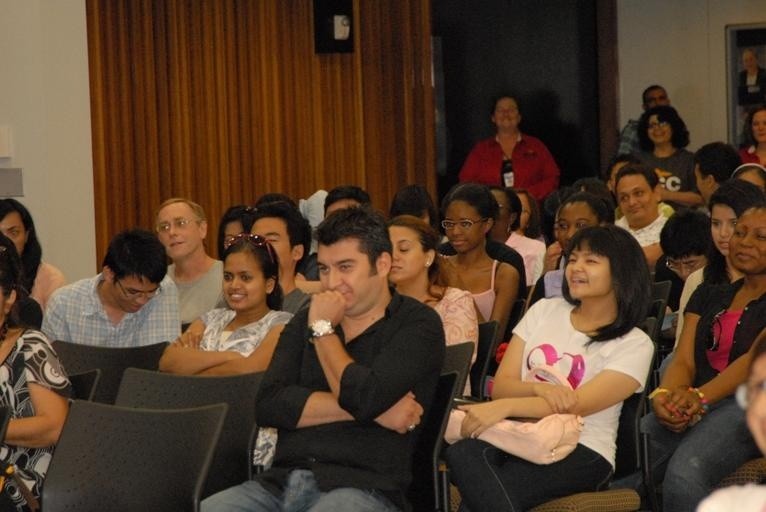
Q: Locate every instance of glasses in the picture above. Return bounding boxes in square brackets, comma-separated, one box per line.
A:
[441, 218, 485, 229]
[156, 218, 200, 233]
[666, 257, 707, 270]
[116, 277, 163, 301]
[228, 233, 273, 265]
[648, 120, 668, 129]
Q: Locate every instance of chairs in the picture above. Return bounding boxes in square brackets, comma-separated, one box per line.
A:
[0, 243, 766, 511]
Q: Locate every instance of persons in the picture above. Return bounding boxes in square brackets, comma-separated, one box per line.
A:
[0, 47, 766, 512]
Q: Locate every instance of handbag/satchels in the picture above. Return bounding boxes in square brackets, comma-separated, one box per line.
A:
[271, 420, 415, 491]
[443, 368, 584, 464]
